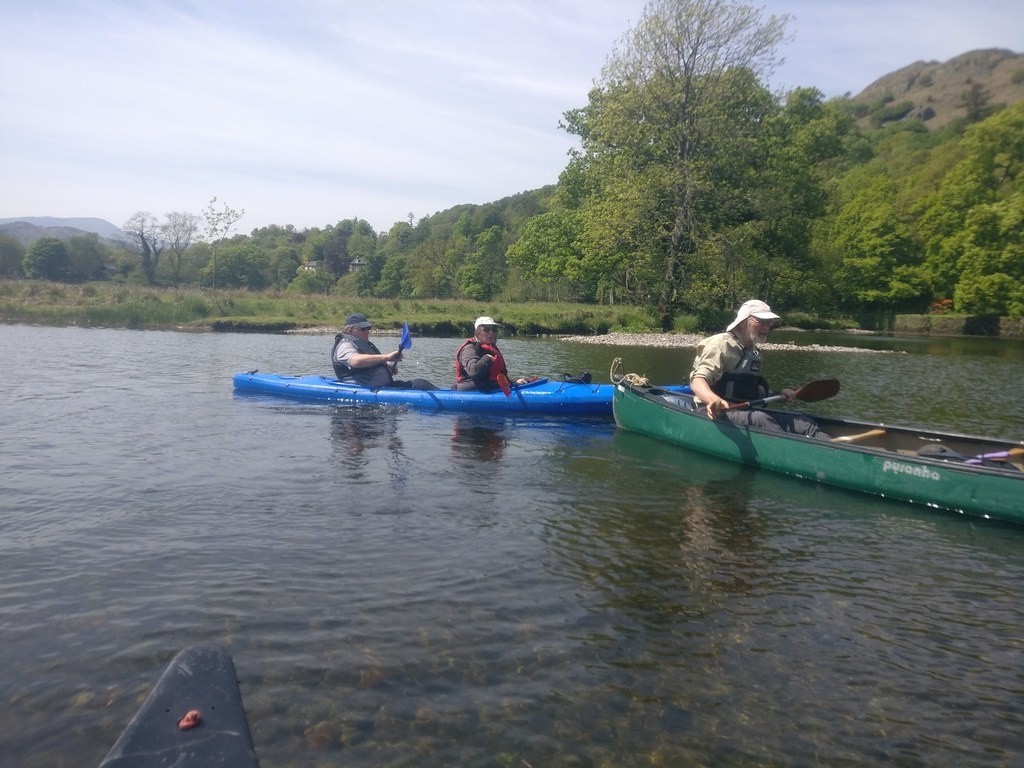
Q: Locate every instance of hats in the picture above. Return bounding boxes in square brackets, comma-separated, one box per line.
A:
[474, 316, 499, 329]
[727, 300, 780, 332]
[344, 313, 373, 329]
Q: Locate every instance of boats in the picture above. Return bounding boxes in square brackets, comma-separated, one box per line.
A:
[98, 642, 260, 767]
[233, 369, 692, 413]
[612, 379, 1024, 526]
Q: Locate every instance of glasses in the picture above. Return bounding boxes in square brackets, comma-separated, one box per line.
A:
[361, 326, 371, 331]
[748, 318, 775, 326]
[483, 326, 498, 333]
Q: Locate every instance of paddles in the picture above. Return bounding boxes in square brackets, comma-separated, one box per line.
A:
[721, 377, 841, 412]
[391, 319, 412, 371]
[496, 374, 539, 397]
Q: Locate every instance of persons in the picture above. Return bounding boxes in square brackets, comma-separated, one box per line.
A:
[331, 312, 442, 390]
[456, 316, 531, 389]
[690, 300, 835, 442]
